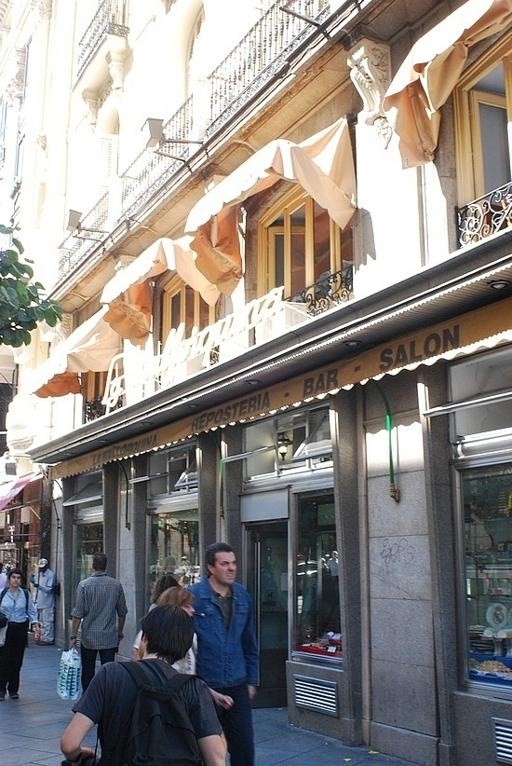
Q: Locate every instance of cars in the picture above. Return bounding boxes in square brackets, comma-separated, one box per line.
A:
[296, 560, 329, 596]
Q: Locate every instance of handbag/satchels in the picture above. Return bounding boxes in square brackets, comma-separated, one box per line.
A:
[57, 651, 83, 701]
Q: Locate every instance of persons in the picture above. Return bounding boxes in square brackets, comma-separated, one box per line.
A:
[0, 570, 43, 701]
[144, 574, 181, 610]
[184, 541, 260, 766]
[31, 558, 56, 646]
[5, 560, 12, 572]
[0, 563, 12, 591]
[68, 553, 128, 696]
[59, 606, 227, 766]
[319, 549, 339, 576]
[141, 587, 233, 766]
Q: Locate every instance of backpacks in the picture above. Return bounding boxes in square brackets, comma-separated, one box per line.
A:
[118, 661, 205, 766]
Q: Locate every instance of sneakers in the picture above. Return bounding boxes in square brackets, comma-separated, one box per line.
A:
[0, 692, 18, 701]
[40, 641, 54, 645]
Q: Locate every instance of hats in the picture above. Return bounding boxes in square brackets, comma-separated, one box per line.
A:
[38, 559, 48, 568]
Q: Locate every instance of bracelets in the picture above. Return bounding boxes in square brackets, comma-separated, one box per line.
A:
[71, 636, 77, 640]
